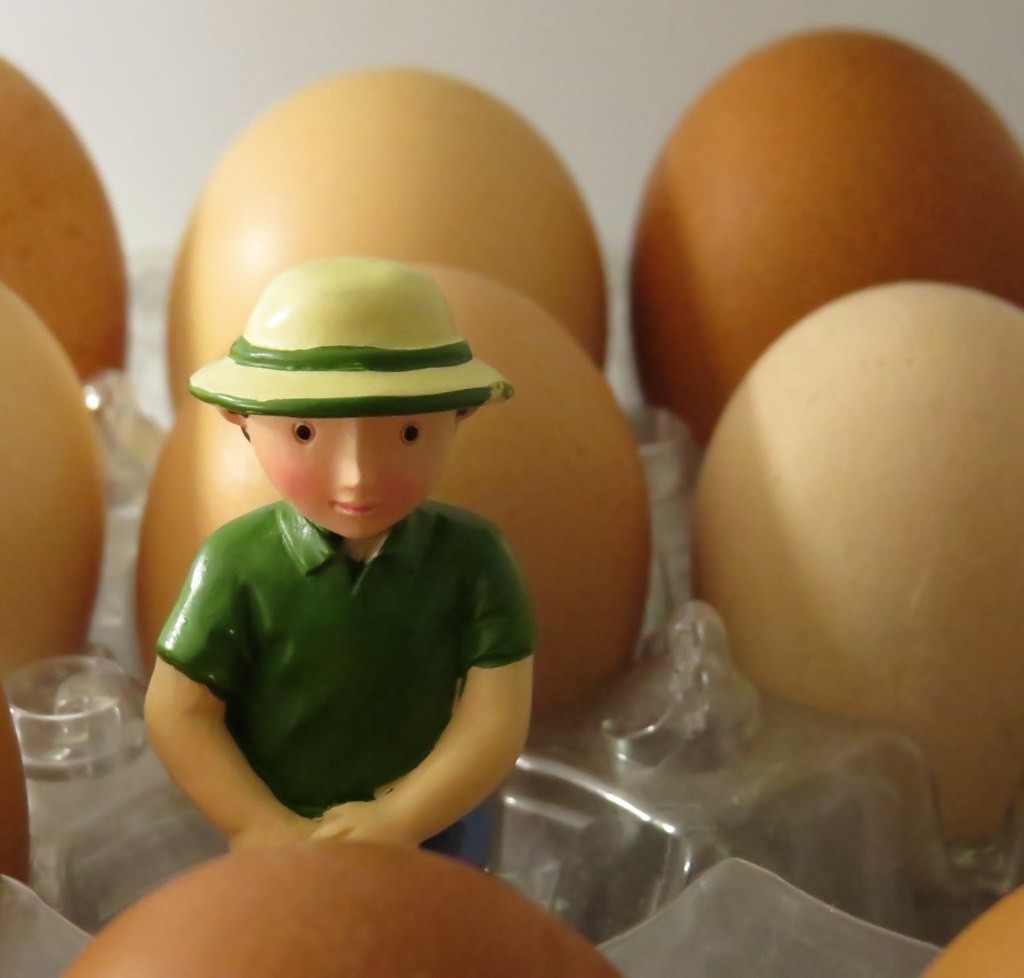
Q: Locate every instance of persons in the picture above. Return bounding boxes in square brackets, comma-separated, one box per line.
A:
[140, 253, 536, 870]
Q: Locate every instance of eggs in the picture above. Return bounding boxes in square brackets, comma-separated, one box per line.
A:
[0, 26, 1024, 978]
